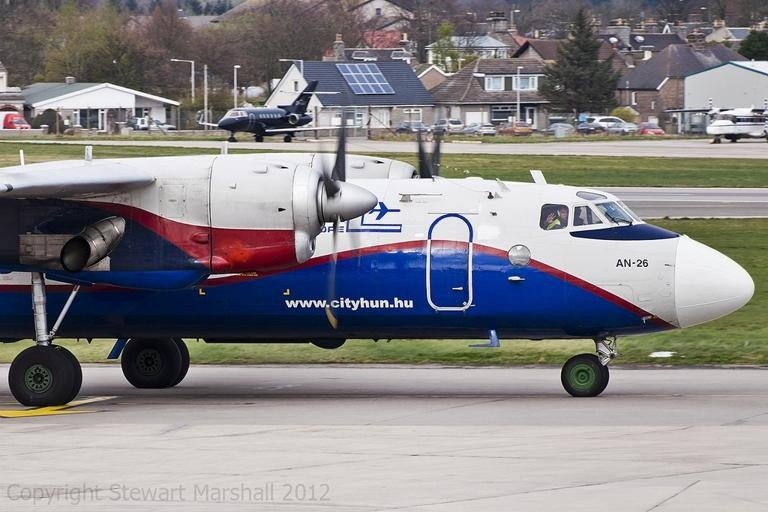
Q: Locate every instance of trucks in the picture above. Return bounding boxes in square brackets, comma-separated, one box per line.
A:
[0, 110, 31, 129]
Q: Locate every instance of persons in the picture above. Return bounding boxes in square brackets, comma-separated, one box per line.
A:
[541, 205, 569, 230]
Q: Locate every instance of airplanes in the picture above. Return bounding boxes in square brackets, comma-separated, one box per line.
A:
[705, 107, 768, 143]
[1, 115, 755, 406]
[198, 79, 362, 142]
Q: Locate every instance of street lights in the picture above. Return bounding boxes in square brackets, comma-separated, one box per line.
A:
[510, 9, 521, 25]
[170, 58, 195, 103]
[233, 65, 241, 108]
[517, 67, 525, 121]
[280, 58, 305, 77]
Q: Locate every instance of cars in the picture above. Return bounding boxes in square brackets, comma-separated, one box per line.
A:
[397, 119, 533, 137]
[542, 116, 664, 135]
[150, 119, 177, 130]
